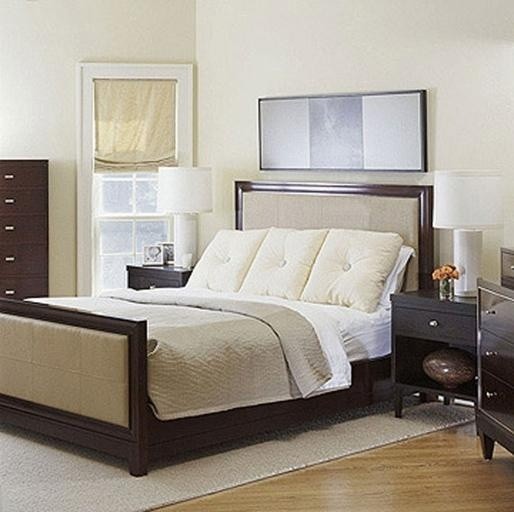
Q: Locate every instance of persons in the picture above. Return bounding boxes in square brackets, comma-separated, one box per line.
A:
[166, 244, 172, 261]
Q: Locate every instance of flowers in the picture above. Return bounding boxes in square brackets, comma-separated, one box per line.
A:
[432, 264, 462, 299]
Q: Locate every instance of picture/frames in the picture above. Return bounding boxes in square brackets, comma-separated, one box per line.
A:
[142, 242, 176, 266]
[257, 88, 427, 173]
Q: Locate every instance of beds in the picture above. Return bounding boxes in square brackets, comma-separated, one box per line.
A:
[1, 180, 434, 478]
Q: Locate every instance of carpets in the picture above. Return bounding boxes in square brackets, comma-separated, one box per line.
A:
[0, 386, 477, 511]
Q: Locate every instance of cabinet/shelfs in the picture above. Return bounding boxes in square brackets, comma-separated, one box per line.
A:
[0, 158, 48, 301]
[474, 247, 514, 459]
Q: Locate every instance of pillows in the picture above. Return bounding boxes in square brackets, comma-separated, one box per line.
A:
[237, 227, 329, 301]
[378, 245, 416, 311]
[185, 229, 267, 294]
[298, 228, 405, 313]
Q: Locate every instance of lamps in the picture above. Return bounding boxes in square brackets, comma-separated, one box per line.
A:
[157, 166, 213, 266]
[431, 171, 506, 298]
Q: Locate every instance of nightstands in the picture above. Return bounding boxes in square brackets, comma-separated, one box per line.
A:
[389, 286, 477, 419]
[125, 263, 193, 291]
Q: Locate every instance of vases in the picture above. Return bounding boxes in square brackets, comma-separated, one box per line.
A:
[439, 278, 454, 302]
